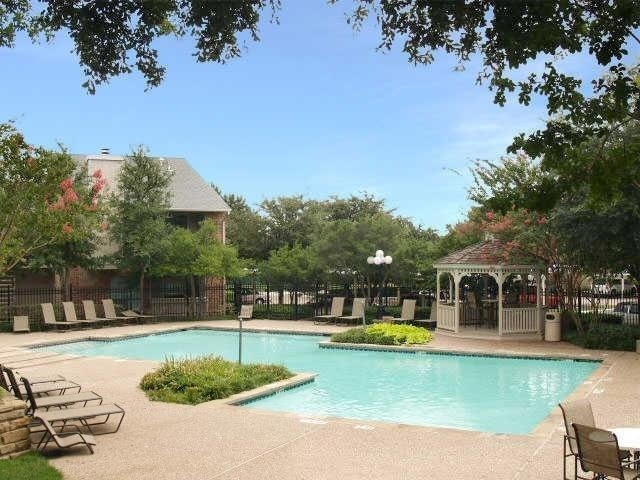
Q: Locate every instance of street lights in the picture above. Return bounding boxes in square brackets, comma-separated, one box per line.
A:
[367, 250, 393, 320]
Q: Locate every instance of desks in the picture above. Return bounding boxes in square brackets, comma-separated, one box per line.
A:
[593, 427, 640, 463]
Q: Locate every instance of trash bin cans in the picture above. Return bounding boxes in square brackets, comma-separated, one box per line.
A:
[544, 311, 561, 342]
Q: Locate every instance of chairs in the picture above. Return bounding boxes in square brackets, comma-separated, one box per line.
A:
[237, 303, 254, 321]
[568, 418, 640, 480]
[557, 398, 597, 480]
[0, 363, 129, 458]
[391, 297, 416, 324]
[311, 297, 348, 329]
[416, 300, 444, 331]
[452, 289, 498, 330]
[10, 296, 157, 336]
[339, 297, 370, 328]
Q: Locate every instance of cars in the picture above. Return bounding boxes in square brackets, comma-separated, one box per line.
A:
[418, 289, 435, 297]
[241, 287, 272, 305]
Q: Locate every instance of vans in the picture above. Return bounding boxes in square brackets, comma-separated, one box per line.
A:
[613, 301, 640, 326]
[591, 277, 637, 298]
